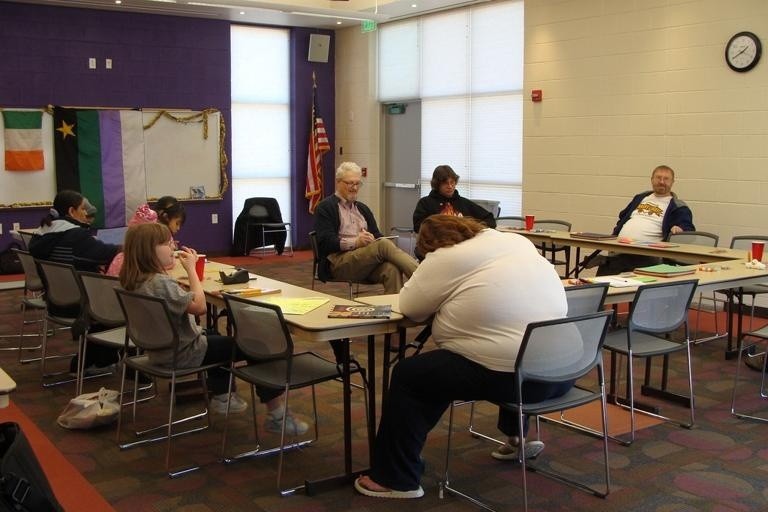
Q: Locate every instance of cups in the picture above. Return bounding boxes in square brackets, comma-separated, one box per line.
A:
[525, 215, 534, 232]
[751, 241, 765, 263]
[195, 254, 206, 282]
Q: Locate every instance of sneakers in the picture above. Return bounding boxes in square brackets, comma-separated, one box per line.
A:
[210, 393, 248, 413]
[264, 411, 308, 436]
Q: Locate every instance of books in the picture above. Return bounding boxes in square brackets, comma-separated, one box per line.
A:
[223, 288, 281, 298]
[634, 264, 697, 277]
[328, 304, 391, 319]
[571, 233, 618, 240]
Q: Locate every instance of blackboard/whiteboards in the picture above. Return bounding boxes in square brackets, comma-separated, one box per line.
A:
[0, 106, 222, 210]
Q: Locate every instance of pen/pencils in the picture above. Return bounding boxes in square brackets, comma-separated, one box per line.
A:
[361, 229, 374, 242]
[622, 276, 637, 279]
[225, 288, 265, 296]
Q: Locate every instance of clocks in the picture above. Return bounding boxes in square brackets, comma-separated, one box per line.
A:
[724, 31, 762, 73]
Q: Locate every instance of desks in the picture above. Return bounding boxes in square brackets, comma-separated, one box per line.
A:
[162, 252, 235, 395]
[173, 269, 403, 498]
[495, 227, 768, 361]
[356, 272, 660, 473]
[622, 260, 768, 409]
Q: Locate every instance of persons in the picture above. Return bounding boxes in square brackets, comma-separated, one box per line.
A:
[354, 215, 583, 498]
[119, 222, 309, 436]
[29, 190, 125, 373]
[596, 166, 695, 276]
[313, 162, 420, 296]
[107, 196, 185, 384]
[413, 165, 496, 262]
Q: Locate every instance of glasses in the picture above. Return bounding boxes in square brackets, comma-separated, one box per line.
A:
[340, 178, 363, 188]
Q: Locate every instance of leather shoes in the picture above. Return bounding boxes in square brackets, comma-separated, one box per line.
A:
[744, 356, 768, 372]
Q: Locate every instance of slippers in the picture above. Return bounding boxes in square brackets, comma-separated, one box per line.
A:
[491, 440, 544, 461]
[355, 476, 425, 498]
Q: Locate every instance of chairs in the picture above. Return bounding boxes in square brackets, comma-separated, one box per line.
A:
[692, 235, 768, 358]
[390, 226, 414, 257]
[442, 281, 615, 512]
[32, 258, 158, 406]
[731, 323, 768, 422]
[0, 230, 78, 363]
[494, 217, 572, 279]
[110, 286, 260, 477]
[307, 230, 397, 301]
[560, 278, 699, 445]
[662, 231, 719, 345]
[242, 197, 293, 260]
[219, 291, 371, 497]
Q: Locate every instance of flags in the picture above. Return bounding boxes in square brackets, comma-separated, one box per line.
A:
[52, 106, 147, 229]
[2, 111, 44, 171]
[305, 86, 331, 214]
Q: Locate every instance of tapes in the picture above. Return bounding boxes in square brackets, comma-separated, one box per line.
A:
[231, 271, 238, 277]
